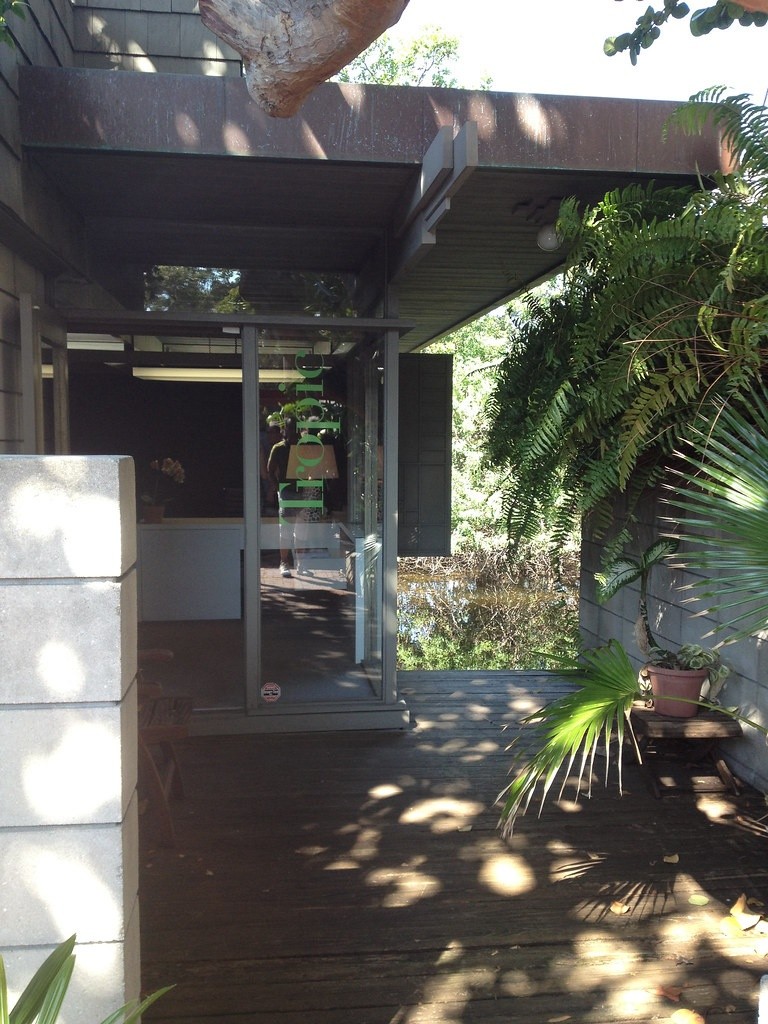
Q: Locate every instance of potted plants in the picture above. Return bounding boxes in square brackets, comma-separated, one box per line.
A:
[644, 642, 736, 717]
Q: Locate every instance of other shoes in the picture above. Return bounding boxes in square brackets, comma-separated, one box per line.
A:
[279, 563, 291, 577]
[297, 564, 304, 575]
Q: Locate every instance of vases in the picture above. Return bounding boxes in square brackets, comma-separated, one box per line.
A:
[142, 505, 165, 524]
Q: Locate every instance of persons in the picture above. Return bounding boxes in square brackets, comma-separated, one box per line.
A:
[267, 415, 341, 578]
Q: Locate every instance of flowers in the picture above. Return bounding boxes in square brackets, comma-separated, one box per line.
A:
[140, 458, 186, 506]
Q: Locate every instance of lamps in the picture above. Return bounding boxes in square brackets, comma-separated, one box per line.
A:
[285, 444, 339, 523]
